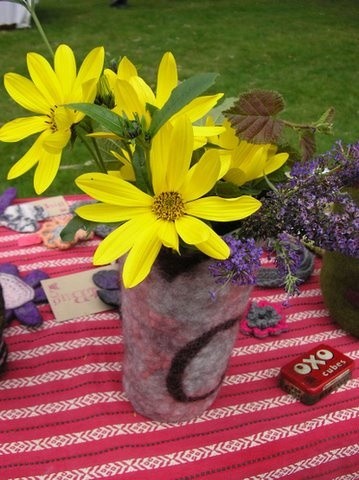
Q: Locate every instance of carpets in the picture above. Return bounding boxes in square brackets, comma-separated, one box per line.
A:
[0, 194, 359, 480]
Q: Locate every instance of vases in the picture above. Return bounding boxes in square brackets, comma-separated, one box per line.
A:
[320, 186, 359, 340]
[120, 259, 250, 423]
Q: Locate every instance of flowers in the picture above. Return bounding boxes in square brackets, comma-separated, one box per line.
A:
[208, 138, 359, 306]
[0, 0, 290, 289]
